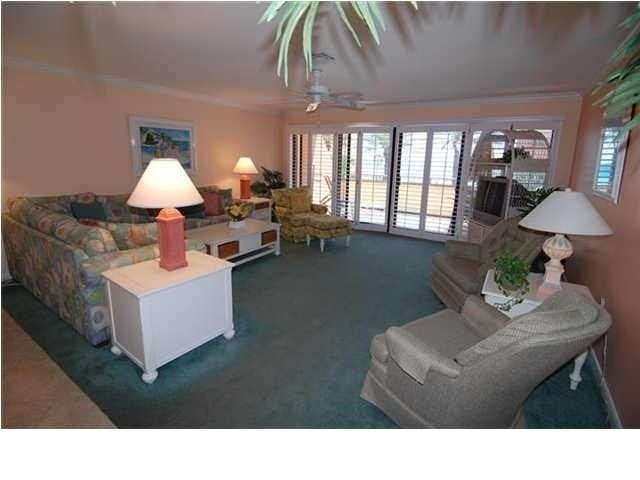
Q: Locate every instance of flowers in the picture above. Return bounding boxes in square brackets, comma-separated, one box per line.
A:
[223, 203, 250, 221]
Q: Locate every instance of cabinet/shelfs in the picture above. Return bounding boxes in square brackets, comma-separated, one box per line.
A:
[468, 218, 494, 244]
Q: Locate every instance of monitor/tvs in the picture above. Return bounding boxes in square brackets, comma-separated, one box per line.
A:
[472, 175, 529, 226]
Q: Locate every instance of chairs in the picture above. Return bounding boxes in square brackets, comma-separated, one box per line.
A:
[270, 185, 329, 244]
[430, 216, 546, 314]
[360, 288, 614, 428]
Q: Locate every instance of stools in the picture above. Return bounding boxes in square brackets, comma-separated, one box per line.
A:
[304, 215, 353, 253]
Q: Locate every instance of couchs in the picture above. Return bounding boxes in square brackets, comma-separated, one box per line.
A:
[1, 183, 233, 347]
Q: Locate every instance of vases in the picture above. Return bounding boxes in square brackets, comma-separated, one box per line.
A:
[228, 221, 245, 228]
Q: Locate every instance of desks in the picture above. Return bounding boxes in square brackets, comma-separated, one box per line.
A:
[99, 249, 237, 385]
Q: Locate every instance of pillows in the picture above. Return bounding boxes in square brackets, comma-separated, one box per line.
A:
[126, 191, 224, 219]
[70, 201, 107, 226]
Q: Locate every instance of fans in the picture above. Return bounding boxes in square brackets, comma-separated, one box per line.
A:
[281, 52, 367, 112]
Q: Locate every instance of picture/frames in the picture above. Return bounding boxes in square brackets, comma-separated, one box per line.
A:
[127, 114, 198, 177]
[592, 87, 635, 206]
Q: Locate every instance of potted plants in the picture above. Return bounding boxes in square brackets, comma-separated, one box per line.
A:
[250, 165, 288, 199]
[491, 246, 532, 313]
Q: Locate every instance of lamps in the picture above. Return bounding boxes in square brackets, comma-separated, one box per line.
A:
[519, 187, 615, 288]
[127, 158, 205, 271]
[232, 157, 259, 200]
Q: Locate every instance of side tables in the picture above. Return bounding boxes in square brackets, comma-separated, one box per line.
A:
[481, 268, 597, 392]
